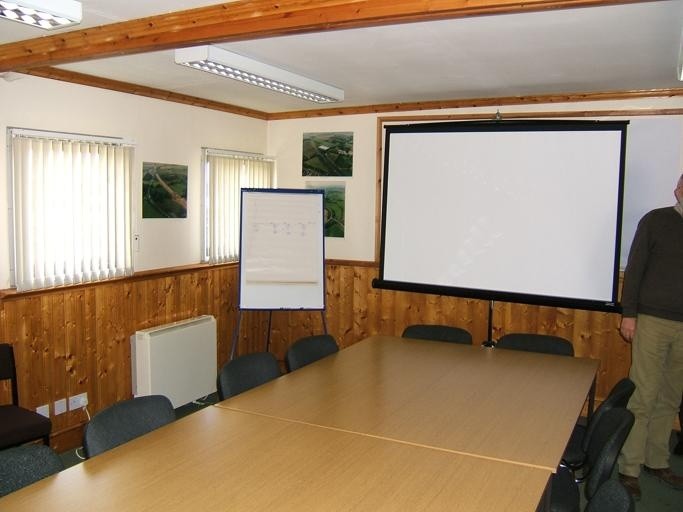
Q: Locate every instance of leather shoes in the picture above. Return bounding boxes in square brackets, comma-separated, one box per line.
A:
[643, 464, 683, 490]
[619, 469, 644, 502]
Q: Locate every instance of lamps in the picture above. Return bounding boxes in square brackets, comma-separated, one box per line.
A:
[173, 42, 345, 105]
[0, 0, 88, 34]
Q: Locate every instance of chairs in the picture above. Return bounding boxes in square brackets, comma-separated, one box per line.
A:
[540, 379, 635, 511]
[285, 334, 340, 373]
[2, 343, 52, 452]
[217, 351, 281, 401]
[81, 395, 176, 460]
[492, 332, 575, 357]
[2, 446, 66, 497]
[400, 324, 473, 346]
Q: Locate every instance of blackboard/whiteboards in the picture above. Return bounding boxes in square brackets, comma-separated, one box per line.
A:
[238, 188, 327, 312]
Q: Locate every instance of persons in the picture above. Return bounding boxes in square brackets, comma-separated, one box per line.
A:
[615, 172, 682, 499]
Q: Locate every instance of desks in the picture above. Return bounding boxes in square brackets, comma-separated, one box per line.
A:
[0, 335, 599, 512]
